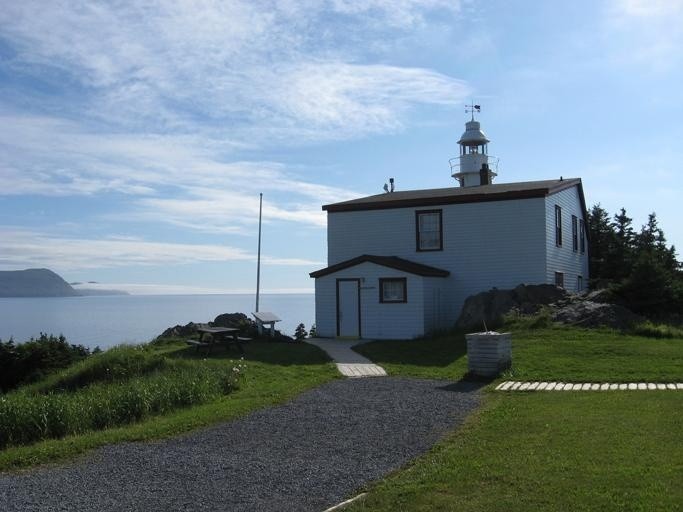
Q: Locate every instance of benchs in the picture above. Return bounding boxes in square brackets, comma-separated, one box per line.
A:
[186, 339, 210, 354]
[225, 335, 252, 349]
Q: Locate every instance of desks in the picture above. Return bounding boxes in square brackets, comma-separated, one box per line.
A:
[197, 327, 241, 352]
